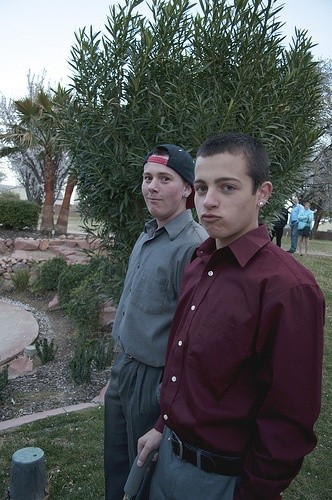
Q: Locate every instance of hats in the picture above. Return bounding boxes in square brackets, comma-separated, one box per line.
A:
[143, 144, 195, 208]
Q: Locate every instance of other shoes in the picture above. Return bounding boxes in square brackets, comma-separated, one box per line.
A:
[286, 250, 295, 253]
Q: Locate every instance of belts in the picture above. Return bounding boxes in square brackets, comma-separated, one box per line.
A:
[168, 430, 257, 476]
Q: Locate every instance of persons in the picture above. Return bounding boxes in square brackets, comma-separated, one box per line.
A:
[286, 197, 304, 253]
[103, 143, 207, 500]
[297, 202, 314, 255]
[269, 207, 288, 248]
[136, 132, 325, 500]
